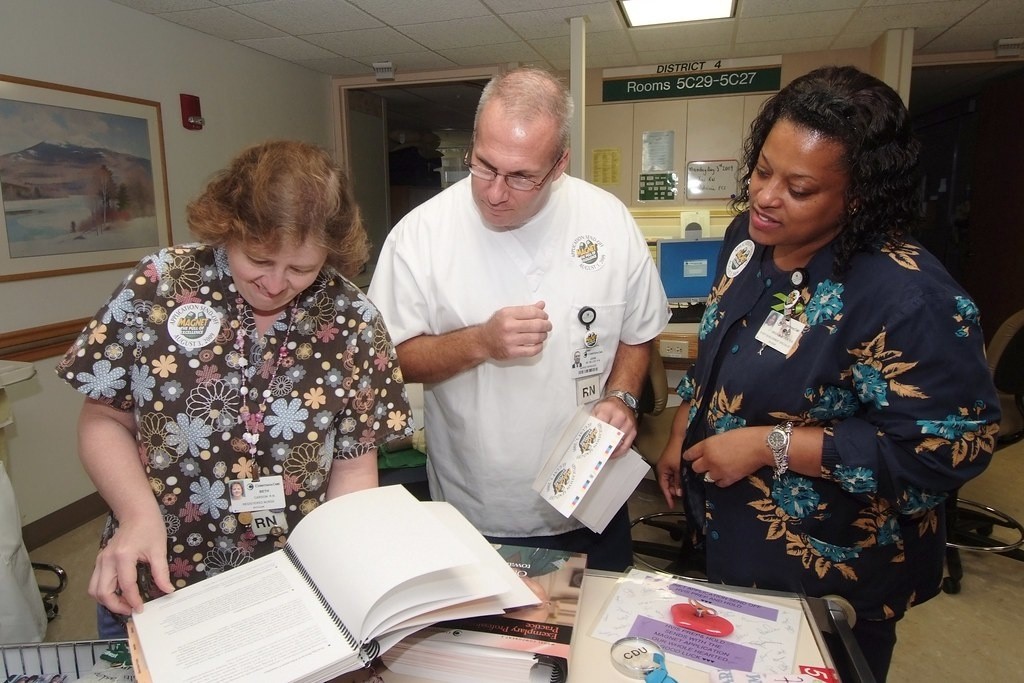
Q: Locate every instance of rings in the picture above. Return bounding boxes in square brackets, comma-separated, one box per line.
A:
[703, 472, 715, 482]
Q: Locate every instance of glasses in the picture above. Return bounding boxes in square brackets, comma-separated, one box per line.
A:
[464, 142, 564, 190]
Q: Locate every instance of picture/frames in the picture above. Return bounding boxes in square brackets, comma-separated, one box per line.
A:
[0, 74, 174, 283]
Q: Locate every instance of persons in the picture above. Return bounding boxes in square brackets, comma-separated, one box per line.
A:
[55, 139, 416, 640]
[765, 313, 778, 327]
[654, 64, 1004, 683]
[496, 545, 572, 624]
[230, 482, 244, 500]
[572, 351, 582, 368]
[367, 62, 674, 573]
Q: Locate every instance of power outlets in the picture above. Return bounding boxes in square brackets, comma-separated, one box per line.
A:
[659, 339, 689, 358]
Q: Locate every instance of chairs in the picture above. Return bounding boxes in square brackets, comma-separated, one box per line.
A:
[943, 311, 1024, 596]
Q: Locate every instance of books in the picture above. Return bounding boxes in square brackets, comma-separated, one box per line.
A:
[127, 483, 541, 682]
[379, 541, 589, 682]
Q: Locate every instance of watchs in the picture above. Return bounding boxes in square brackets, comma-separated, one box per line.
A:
[605, 389, 640, 413]
[766, 420, 793, 476]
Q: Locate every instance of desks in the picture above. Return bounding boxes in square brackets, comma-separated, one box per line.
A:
[586, 567, 872, 683]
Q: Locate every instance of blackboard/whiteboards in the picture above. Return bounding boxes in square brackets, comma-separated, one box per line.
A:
[686, 159, 740, 200]
